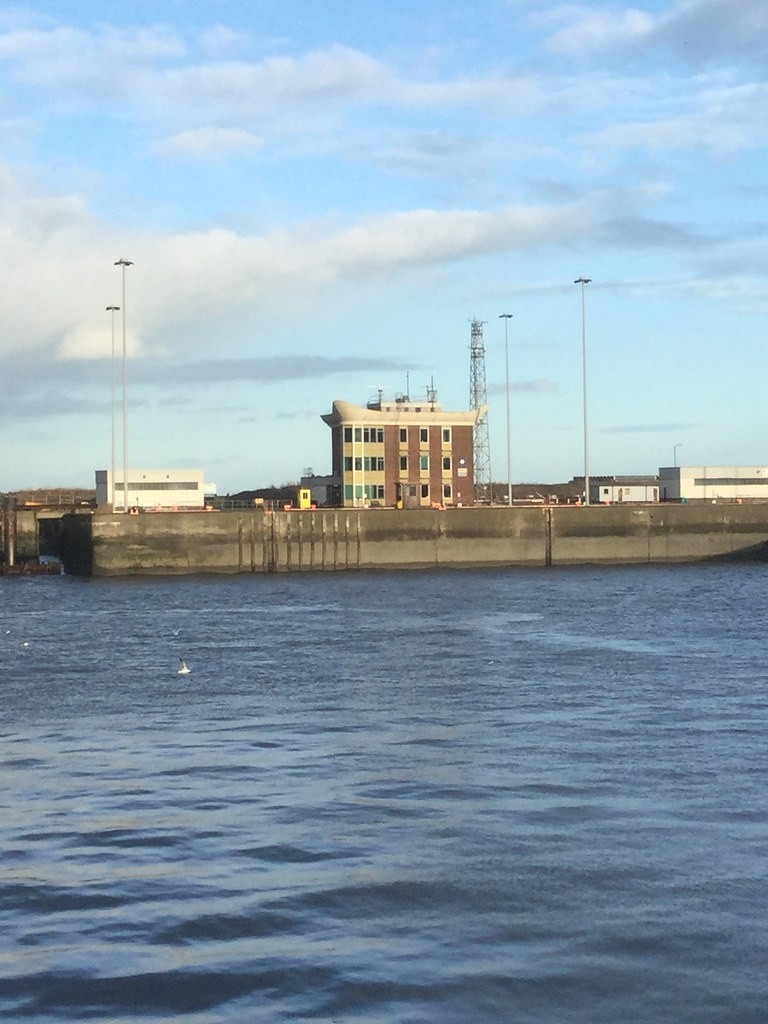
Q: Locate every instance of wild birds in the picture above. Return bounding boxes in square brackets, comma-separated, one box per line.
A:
[178, 658, 190, 675]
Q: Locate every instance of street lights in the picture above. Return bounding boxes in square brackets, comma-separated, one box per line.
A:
[106, 304, 120, 514]
[114, 256, 136, 515]
[673, 443, 683, 467]
[575, 276, 592, 506]
[498, 313, 515, 505]
